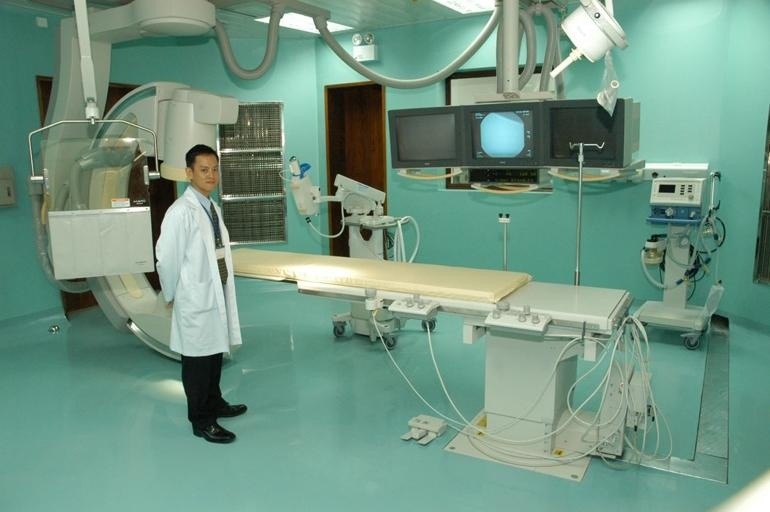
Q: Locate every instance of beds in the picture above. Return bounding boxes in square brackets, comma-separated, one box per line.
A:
[231, 247, 532, 316]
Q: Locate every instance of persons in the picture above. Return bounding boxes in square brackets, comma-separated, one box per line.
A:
[155, 144, 247, 443]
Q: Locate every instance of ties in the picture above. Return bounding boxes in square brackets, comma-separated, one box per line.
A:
[210, 201, 228, 285]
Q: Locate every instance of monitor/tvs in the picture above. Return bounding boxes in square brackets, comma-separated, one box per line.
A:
[388, 106, 466, 169]
[465, 102, 545, 169]
[544, 97, 640, 170]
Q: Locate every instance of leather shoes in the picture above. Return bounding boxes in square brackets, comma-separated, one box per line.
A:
[216, 405, 247, 417]
[193, 422, 235, 443]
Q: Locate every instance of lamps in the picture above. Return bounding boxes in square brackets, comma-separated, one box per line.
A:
[348, 32, 377, 62]
